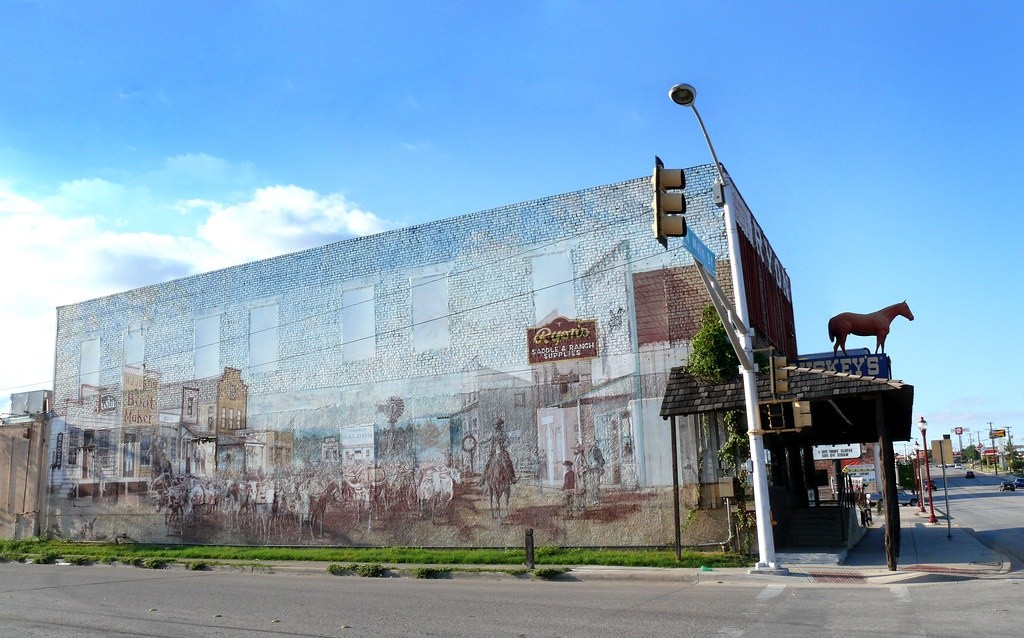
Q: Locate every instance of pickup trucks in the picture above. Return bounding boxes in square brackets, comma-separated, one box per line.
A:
[867, 487, 919, 508]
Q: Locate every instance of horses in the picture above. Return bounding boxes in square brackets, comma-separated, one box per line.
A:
[827, 299, 915, 357]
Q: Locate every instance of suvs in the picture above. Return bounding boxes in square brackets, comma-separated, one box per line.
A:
[924, 480, 937, 492]
[966, 471, 974, 479]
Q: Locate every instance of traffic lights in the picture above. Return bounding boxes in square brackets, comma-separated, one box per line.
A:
[650, 155, 691, 252]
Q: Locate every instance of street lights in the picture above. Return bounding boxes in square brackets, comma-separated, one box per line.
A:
[918, 416, 941, 526]
[667, 82, 790, 576]
[910, 451, 919, 495]
[914, 441, 927, 513]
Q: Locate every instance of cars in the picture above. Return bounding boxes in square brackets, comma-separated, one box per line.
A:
[955, 463, 962, 469]
[999, 481, 1015, 492]
[1015, 479, 1024, 489]
[937, 463, 955, 467]
